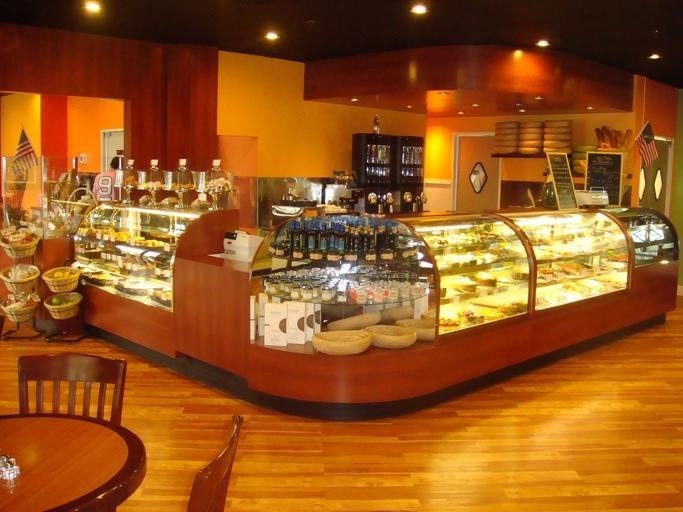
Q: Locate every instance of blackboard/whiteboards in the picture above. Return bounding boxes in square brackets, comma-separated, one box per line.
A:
[546, 152, 579, 211]
[584, 151, 623, 208]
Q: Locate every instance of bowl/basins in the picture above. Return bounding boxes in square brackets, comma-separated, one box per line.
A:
[0, 231, 82, 323]
[494, 120, 572, 156]
[311, 304, 437, 355]
[571, 145, 597, 174]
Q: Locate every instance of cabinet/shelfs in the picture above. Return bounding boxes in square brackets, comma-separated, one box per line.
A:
[73, 194, 241, 367]
[589, 202, 679, 335]
[484, 198, 634, 363]
[258, 215, 425, 413]
[352, 130, 423, 190]
[404, 210, 532, 392]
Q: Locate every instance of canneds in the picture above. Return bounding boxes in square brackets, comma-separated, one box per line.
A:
[262, 274, 340, 303]
[269, 240, 420, 265]
[79, 231, 138, 272]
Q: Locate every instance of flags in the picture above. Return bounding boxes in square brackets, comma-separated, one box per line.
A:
[636, 122, 659, 169]
[11, 128, 40, 176]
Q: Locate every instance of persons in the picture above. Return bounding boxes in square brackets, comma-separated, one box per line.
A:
[92, 157, 123, 202]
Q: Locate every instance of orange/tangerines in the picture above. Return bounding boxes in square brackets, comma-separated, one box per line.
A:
[52, 298, 60, 304]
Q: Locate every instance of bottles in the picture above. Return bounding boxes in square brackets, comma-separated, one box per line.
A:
[287, 216, 400, 251]
[366, 144, 422, 165]
[0, 451, 21, 486]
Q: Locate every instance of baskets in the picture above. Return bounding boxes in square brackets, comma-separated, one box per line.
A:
[326, 310, 381, 331]
[397, 316, 436, 341]
[381, 303, 415, 324]
[41, 265, 79, 293]
[1, 228, 41, 259]
[43, 292, 83, 320]
[1, 264, 39, 292]
[365, 325, 415, 349]
[422, 309, 437, 320]
[0, 292, 39, 323]
[313, 329, 372, 354]
[494, 119, 574, 156]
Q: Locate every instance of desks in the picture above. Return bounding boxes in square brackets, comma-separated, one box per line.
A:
[2, 413, 149, 512]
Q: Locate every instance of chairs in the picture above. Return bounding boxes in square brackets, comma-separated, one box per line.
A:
[187, 412, 244, 512]
[19, 351, 129, 426]
